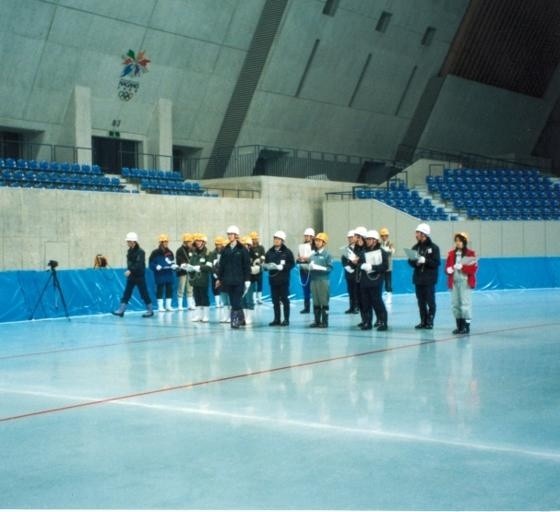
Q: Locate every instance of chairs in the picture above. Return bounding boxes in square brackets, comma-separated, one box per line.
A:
[123, 166, 220, 197]
[424, 166, 560, 220]
[1, 156, 138, 194]
[353, 179, 458, 222]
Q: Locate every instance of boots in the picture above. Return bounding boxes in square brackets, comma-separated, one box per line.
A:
[112, 304, 127, 317]
[157, 299, 166, 311]
[358, 311, 365, 327]
[142, 303, 153, 316]
[258, 292, 264, 304]
[217, 305, 227, 322]
[374, 308, 381, 328]
[252, 292, 258, 305]
[311, 304, 322, 327]
[353, 296, 359, 313]
[269, 304, 281, 325]
[426, 303, 435, 329]
[190, 306, 202, 322]
[282, 305, 289, 326]
[215, 295, 220, 308]
[322, 305, 329, 328]
[231, 310, 239, 329]
[345, 295, 353, 313]
[415, 308, 426, 328]
[178, 297, 185, 310]
[452, 318, 462, 334]
[201, 306, 210, 322]
[219, 295, 223, 307]
[187, 296, 196, 309]
[384, 293, 392, 306]
[361, 309, 372, 330]
[238, 310, 245, 326]
[225, 307, 231, 322]
[377, 311, 387, 330]
[460, 319, 470, 334]
[166, 298, 174, 311]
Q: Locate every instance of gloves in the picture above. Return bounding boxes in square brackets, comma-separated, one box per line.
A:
[311, 265, 326, 271]
[187, 264, 194, 272]
[361, 263, 372, 272]
[156, 264, 162, 271]
[275, 265, 284, 270]
[244, 280, 252, 288]
[416, 261, 421, 266]
[308, 261, 314, 269]
[124, 270, 131, 276]
[447, 267, 454, 274]
[345, 265, 355, 273]
[181, 262, 188, 270]
[454, 264, 463, 270]
[193, 265, 201, 273]
[171, 264, 178, 270]
[253, 258, 260, 266]
[418, 256, 425, 263]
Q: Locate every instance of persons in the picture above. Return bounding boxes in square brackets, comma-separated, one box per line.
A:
[307, 232, 334, 328]
[176, 225, 266, 328]
[111, 232, 154, 318]
[262, 230, 296, 327]
[379, 227, 395, 292]
[352, 226, 381, 327]
[340, 229, 359, 315]
[444, 230, 479, 335]
[295, 226, 316, 314]
[352, 229, 390, 332]
[148, 232, 179, 312]
[407, 222, 441, 329]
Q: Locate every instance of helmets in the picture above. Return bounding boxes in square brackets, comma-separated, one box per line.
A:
[354, 227, 367, 236]
[240, 236, 253, 245]
[304, 228, 315, 236]
[380, 228, 388, 236]
[159, 233, 169, 241]
[181, 233, 192, 241]
[195, 234, 208, 242]
[313, 233, 329, 242]
[125, 232, 138, 241]
[273, 231, 287, 241]
[227, 225, 240, 235]
[415, 223, 431, 235]
[215, 236, 225, 245]
[348, 230, 354, 237]
[249, 231, 258, 239]
[365, 230, 380, 239]
[192, 232, 201, 240]
[454, 230, 468, 240]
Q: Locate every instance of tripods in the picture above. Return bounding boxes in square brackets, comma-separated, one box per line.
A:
[28, 272, 70, 322]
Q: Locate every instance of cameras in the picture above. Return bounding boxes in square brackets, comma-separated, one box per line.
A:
[48, 260, 58, 271]
